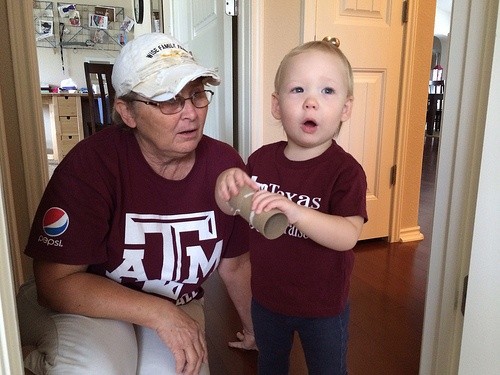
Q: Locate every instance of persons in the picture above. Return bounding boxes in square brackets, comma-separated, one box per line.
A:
[214, 40, 367, 375]
[16, 34, 257, 374]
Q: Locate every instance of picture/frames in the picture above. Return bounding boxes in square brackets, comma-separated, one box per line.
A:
[150, 0, 164, 33]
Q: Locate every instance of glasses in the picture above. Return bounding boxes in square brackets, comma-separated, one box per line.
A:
[131, 90, 214, 115]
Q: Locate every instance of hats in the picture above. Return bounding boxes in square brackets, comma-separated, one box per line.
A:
[112, 32, 221, 101]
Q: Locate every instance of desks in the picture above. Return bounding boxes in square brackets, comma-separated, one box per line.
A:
[42, 92, 103, 164]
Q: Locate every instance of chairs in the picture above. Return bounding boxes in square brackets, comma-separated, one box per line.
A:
[84, 62, 113, 134]
[427, 79, 443, 133]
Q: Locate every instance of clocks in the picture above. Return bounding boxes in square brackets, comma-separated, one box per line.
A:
[135, 0, 143, 23]
